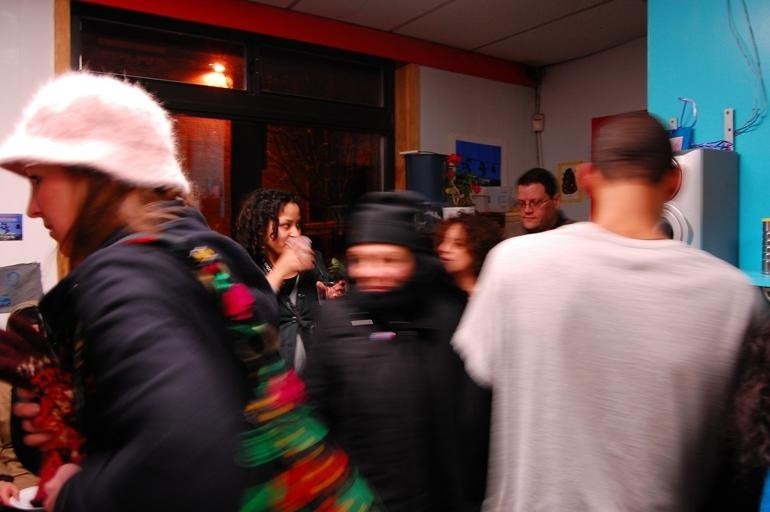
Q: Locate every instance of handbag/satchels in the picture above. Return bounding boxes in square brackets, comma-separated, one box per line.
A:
[236, 368, 387, 512]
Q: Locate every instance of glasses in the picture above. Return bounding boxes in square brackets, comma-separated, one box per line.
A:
[513, 197, 552, 210]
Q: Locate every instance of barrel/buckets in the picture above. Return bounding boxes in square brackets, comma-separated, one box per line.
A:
[400, 149, 447, 202]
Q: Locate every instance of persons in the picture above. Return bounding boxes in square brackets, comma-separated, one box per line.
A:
[237, 189, 346, 380]
[0, 462, 21, 508]
[507, 168, 576, 238]
[450, 113, 770, 512]
[301, 190, 489, 512]
[436, 211, 504, 300]
[0, 69, 389, 511]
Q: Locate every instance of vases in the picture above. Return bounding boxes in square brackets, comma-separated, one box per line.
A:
[442, 208, 475, 223]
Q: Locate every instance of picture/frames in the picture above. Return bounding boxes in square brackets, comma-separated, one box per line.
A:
[557, 161, 585, 204]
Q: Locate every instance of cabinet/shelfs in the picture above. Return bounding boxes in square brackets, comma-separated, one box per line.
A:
[481, 213, 527, 238]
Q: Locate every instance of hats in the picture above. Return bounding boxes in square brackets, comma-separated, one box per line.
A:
[1, 71, 189, 197]
[341, 192, 442, 256]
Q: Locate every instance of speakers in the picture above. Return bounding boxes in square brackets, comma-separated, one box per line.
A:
[659, 148, 740, 270]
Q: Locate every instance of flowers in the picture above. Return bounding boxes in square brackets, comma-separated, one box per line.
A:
[443, 153, 481, 207]
